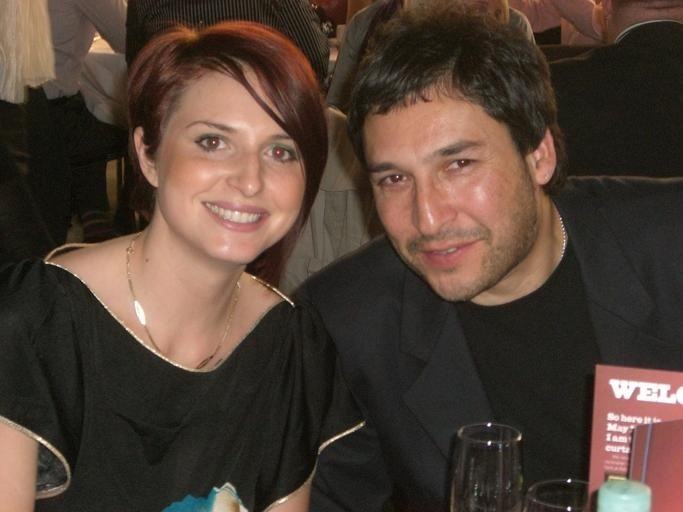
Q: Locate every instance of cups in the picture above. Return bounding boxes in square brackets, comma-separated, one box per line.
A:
[448, 423, 524, 512]
[523, 477, 597, 512]
